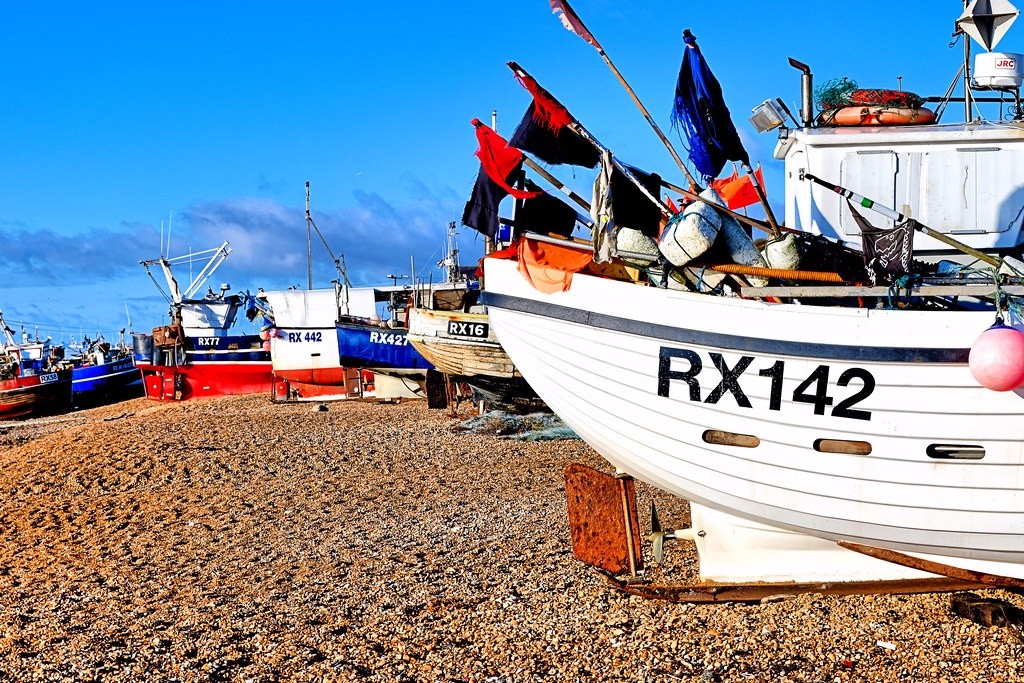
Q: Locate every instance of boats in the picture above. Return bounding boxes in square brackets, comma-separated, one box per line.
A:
[483, 0, 1024, 579]
[0, 183, 539, 422]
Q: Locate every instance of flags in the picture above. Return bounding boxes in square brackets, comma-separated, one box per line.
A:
[476, 125, 539, 199]
[712, 172, 738, 197]
[721, 169, 766, 209]
[610, 171, 661, 236]
[461, 160, 522, 237]
[507, 100, 600, 169]
[514, 185, 577, 242]
[846, 199, 913, 281]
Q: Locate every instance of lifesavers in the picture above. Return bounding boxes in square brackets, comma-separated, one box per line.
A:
[815, 89, 936, 126]
